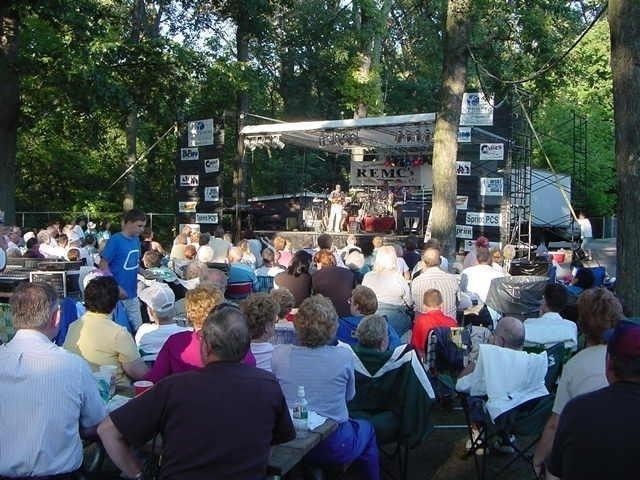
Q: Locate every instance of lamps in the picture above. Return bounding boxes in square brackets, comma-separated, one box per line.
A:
[318, 127, 363, 146]
[393, 124, 433, 143]
[381, 155, 434, 167]
[242, 134, 286, 153]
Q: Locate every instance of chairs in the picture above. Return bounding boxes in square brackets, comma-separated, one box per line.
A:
[0, 245, 611, 477]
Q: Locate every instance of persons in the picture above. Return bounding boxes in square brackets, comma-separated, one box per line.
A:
[0, 209, 639, 480]
[325, 184, 345, 233]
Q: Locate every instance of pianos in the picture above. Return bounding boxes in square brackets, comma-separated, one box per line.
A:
[396, 198, 432, 235]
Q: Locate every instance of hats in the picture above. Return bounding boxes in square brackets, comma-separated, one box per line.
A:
[603, 319, 640, 359]
[139, 284, 176, 311]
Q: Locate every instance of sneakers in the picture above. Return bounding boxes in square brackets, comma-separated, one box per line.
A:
[466, 436, 515, 456]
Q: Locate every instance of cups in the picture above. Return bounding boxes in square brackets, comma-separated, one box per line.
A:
[133, 380, 154, 395]
[91, 365, 118, 404]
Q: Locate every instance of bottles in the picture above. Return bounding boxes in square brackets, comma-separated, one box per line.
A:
[292, 385, 309, 439]
